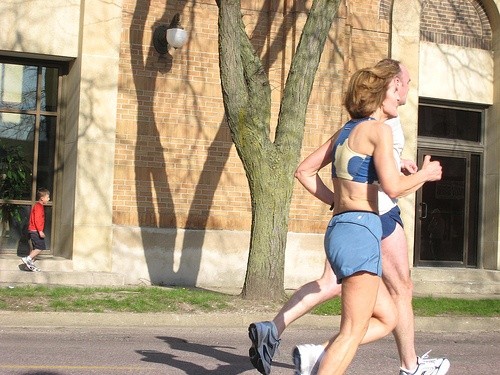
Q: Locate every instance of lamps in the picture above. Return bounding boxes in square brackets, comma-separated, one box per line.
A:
[153, 12, 189, 55]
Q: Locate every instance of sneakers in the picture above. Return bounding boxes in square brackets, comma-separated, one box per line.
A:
[21, 257, 41, 272]
[247, 320, 282, 375]
[399, 350, 450, 375]
[292, 343, 326, 375]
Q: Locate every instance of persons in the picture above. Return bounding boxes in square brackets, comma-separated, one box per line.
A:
[291, 69, 442, 375]
[248, 57, 450, 375]
[21, 187, 50, 272]
